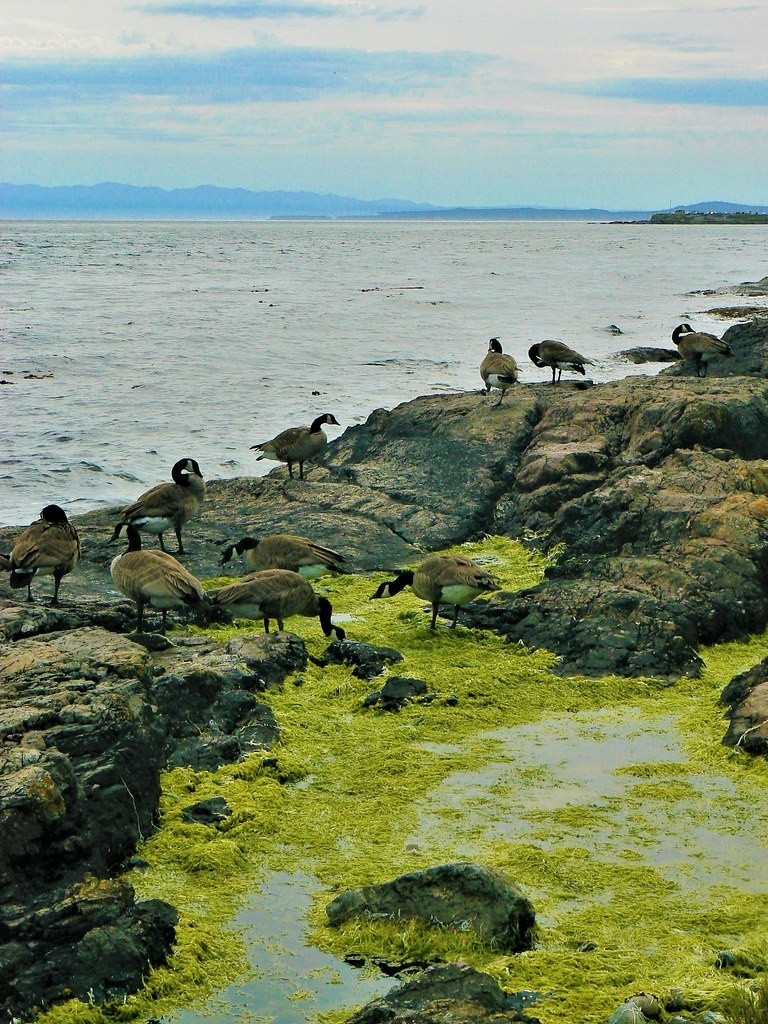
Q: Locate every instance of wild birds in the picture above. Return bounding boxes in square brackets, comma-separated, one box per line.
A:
[249, 414, 341, 482]
[120, 457, 206, 556]
[106, 522, 214, 637]
[529, 339, 596, 386]
[478, 338, 523, 408]
[6, 504, 82, 608]
[217, 534, 353, 576]
[207, 568, 349, 642]
[672, 324, 736, 379]
[369, 554, 503, 630]
